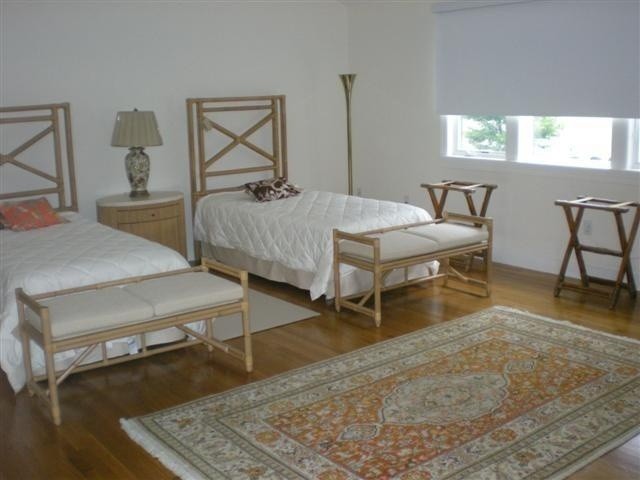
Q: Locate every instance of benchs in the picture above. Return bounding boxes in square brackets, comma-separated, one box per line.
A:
[331, 212, 493, 328]
[14, 257, 255, 424]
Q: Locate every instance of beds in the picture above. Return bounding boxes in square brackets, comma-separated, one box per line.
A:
[186, 94, 439, 307]
[0, 101, 215, 394]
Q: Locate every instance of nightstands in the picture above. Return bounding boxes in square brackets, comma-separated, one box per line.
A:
[96, 190, 187, 260]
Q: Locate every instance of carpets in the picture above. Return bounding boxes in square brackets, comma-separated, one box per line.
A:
[117, 300, 640, 480]
[212, 286, 322, 343]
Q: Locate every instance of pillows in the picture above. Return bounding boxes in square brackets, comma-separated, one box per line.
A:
[0, 196, 71, 232]
[244, 175, 301, 203]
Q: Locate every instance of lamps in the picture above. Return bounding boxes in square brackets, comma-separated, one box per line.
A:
[110, 107, 164, 199]
